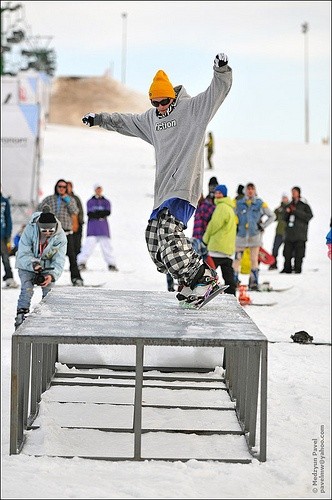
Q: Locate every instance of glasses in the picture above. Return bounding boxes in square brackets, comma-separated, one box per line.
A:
[151, 98, 171, 107]
[41, 229, 55, 233]
[57, 185, 67, 188]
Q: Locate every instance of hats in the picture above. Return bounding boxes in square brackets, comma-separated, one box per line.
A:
[148, 70, 175, 99]
[214, 185, 227, 197]
[209, 177, 218, 185]
[37, 204, 57, 228]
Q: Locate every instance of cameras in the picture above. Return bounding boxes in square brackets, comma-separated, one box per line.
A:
[31, 272, 45, 287]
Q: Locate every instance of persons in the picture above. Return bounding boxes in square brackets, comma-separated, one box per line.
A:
[67, 182, 84, 281]
[280, 187, 313, 273]
[37, 179, 81, 285]
[14, 204, 67, 334]
[200, 186, 239, 296]
[9, 224, 26, 256]
[1, 193, 18, 288]
[234, 183, 276, 291]
[192, 177, 219, 242]
[269, 193, 289, 270]
[77, 183, 119, 271]
[81, 52, 232, 311]
[205, 132, 214, 169]
[236, 185, 245, 200]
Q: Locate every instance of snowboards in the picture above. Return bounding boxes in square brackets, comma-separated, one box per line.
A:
[258, 247, 275, 265]
[197, 285, 230, 310]
[58, 283, 105, 287]
[259, 287, 292, 292]
[249, 303, 277, 306]
[266, 332, 330, 345]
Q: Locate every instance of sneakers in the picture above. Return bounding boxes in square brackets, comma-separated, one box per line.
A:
[5, 278, 18, 288]
[71, 278, 83, 286]
[176, 285, 193, 301]
[187, 268, 219, 307]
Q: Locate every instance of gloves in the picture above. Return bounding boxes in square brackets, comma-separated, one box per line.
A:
[82, 113, 95, 127]
[214, 53, 228, 67]
